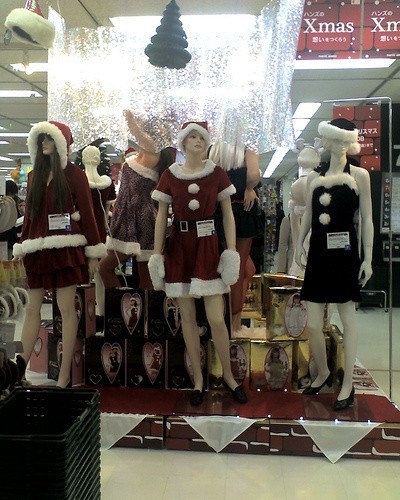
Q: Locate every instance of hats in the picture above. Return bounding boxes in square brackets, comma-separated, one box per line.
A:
[177, 122, 209, 151]
[317, 117, 357, 142]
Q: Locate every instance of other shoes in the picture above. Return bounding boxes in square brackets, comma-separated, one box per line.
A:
[221, 376, 247, 403]
[190, 387, 203, 405]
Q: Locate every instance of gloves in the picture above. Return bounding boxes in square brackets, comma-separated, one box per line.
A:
[217, 249, 240, 285]
[147, 254, 164, 291]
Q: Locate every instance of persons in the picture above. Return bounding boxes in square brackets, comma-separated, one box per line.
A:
[149, 347, 161, 377]
[295, 118, 375, 411]
[267, 348, 286, 387]
[109, 347, 120, 372]
[151, 119, 247, 403]
[13, 120, 104, 388]
[74, 295, 80, 320]
[210, 106, 260, 328]
[127, 296, 143, 329]
[97, 105, 177, 287]
[289, 145, 320, 274]
[79, 144, 116, 315]
[166, 296, 176, 329]
[230, 345, 243, 377]
[289, 294, 304, 337]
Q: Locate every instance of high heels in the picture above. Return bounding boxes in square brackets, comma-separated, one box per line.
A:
[335, 386, 355, 410]
[302, 372, 333, 396]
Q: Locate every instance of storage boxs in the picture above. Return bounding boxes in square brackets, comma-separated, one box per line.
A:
[0, 387, 103, 500]
